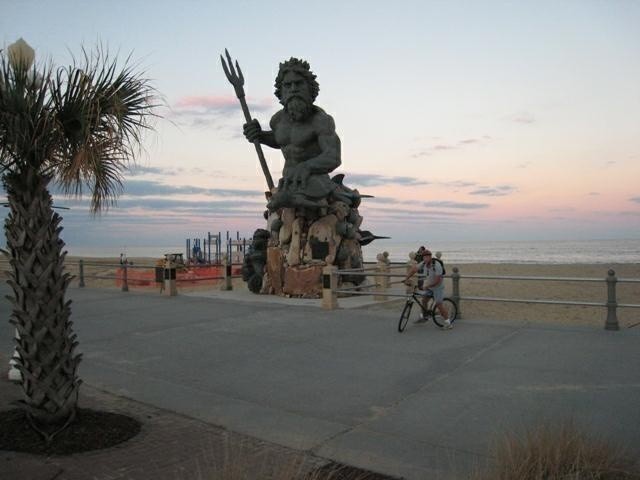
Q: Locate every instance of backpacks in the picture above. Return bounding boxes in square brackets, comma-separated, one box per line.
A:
[431, 258, 447, 275]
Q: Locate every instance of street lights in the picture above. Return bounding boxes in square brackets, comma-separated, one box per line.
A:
[1, 32, 45, 176]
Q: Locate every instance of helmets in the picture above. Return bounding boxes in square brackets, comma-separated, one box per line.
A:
[422, 250, 433, 257]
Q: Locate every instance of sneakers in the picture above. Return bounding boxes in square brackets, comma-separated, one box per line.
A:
[413, 317, 428, 325]
[440, 322, 454, 331]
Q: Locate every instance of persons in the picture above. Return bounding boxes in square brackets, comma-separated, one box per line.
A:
[243, 57, 342, 191]
[414, 246, 425, 290]
[405, 249, 452, 331]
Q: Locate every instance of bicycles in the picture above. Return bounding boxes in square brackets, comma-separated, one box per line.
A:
[396, 278, 458, 336]
